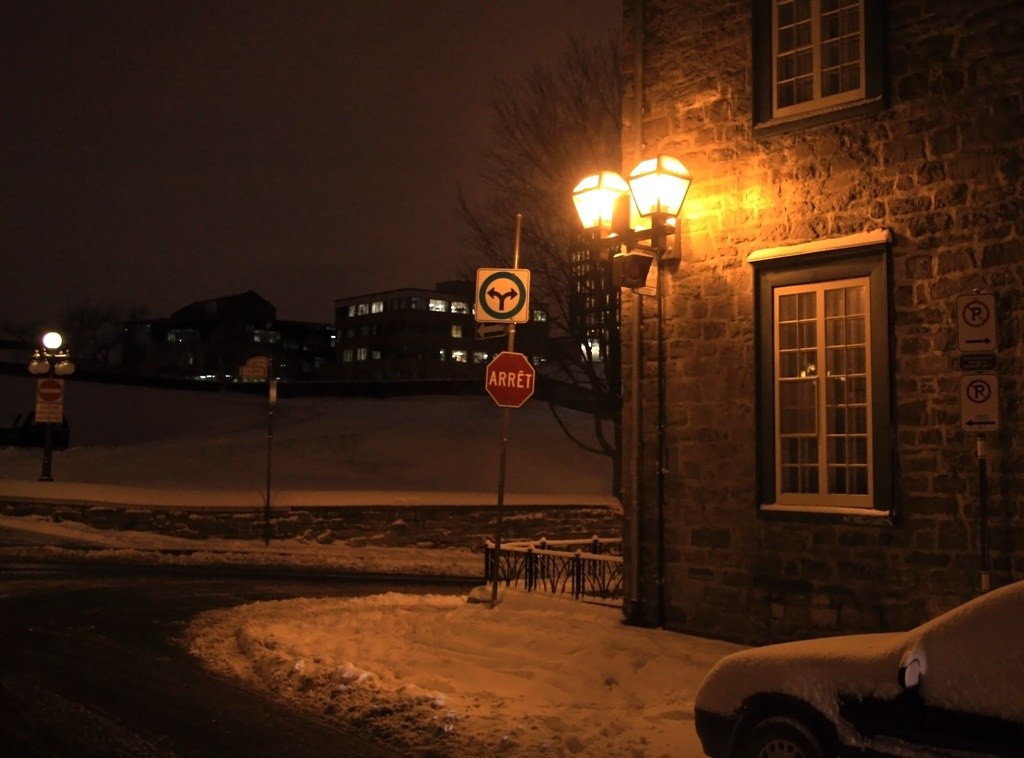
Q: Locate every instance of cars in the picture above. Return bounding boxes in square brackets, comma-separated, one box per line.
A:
[692, 577, 1024, 758]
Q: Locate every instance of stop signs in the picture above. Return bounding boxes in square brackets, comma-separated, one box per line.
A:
[38, 379, 63, 403]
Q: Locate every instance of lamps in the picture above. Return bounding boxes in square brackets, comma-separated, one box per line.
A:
[572, 155, 690, 264]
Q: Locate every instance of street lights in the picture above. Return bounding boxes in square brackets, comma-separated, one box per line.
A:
[28, 331, 76, 482]
